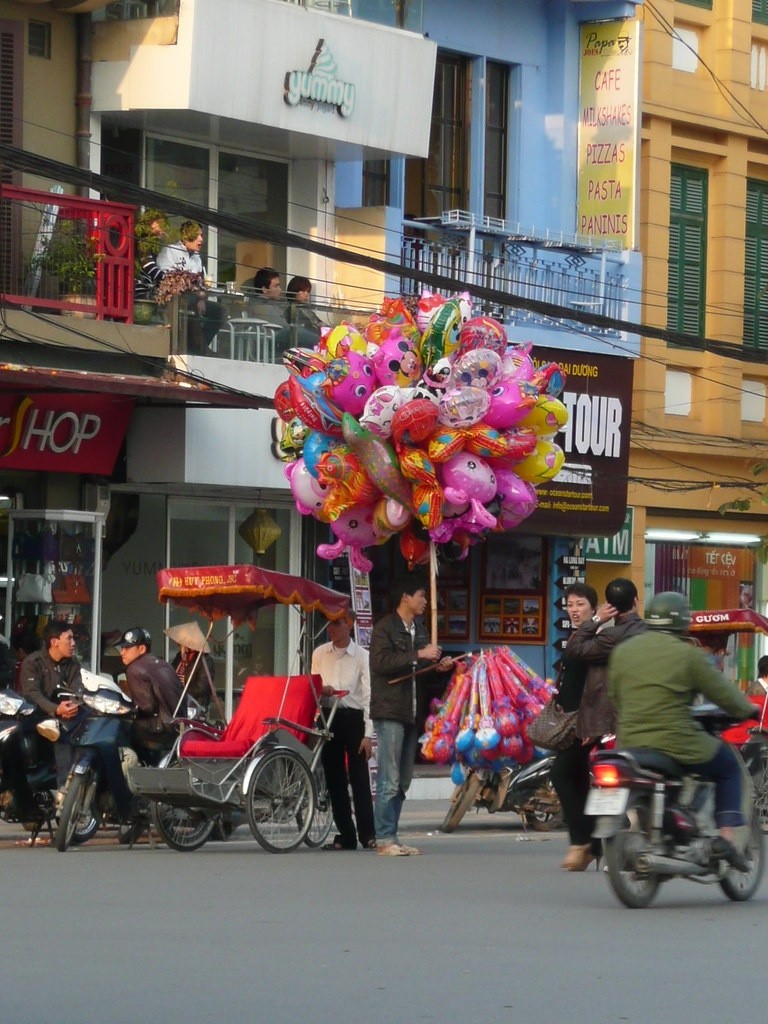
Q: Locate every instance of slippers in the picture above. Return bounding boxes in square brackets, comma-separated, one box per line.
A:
[401, 844, 423, 856]
[378, 845, 410, 857]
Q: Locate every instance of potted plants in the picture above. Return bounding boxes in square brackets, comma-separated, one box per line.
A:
[28, 219, 107, 321]
[132, 177, 199, 323]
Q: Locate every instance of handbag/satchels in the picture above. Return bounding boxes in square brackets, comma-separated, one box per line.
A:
[526, 699, 580, 751]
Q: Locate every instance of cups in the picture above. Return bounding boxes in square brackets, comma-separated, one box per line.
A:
[226, 281, 236, 294]
[204, 274, 212, 285]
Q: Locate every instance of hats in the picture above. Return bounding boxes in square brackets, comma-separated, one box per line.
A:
[162, 621, 211, 653]
[112, 627, 152, 647]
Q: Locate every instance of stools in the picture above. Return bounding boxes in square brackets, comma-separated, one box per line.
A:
[209, 315, 283, 365]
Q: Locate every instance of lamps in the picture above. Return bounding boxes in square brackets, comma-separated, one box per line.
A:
[645, 529, 761, 541]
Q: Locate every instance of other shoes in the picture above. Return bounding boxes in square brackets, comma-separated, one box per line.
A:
[37, 719, 60, 742]
[711, 836, 748, 873]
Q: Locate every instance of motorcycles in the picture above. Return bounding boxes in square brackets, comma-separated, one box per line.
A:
[441, 746, 564, 834]
[0, 685, 234, 852]
[583, 702, 766, 908]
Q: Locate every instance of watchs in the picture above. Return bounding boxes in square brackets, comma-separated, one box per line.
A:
[592, 615, 602, 625]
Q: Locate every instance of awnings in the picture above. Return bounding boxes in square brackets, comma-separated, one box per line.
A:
[0, 361, 275, 410]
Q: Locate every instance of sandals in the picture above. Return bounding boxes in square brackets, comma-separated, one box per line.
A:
[364, 839, 379, 850]
[321, 841, 345, 850]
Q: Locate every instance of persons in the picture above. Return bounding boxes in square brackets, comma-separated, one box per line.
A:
[138, 210, 331, 362]
[608, 590, 762, 871]
[743, 655, 768, 696]
[16, 620, 215, 843]
[547, 584, 603, 871]
[367, 579, 454, 856]
[561, 576, 647, 732]
[311, 608, 376, 851]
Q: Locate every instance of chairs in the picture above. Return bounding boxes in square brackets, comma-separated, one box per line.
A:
[175, 672, 322, 769]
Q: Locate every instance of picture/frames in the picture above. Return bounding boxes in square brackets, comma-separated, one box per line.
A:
[481, 594, 542, 637]
[419, 549, 471, 642]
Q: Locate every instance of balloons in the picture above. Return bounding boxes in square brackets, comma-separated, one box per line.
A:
[419, 646, 559, 786]
[272, 290, 567, 572]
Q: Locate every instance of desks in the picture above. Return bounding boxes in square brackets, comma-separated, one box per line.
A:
[205, 287, 269, 361]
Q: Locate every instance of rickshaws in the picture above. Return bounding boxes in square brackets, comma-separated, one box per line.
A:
[128, 565, 351, 854]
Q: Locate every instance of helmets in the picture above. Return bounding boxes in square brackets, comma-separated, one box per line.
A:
[644, 591, 690, 629]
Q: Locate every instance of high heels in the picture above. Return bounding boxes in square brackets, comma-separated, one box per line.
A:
[562, 842, 603, 872]
